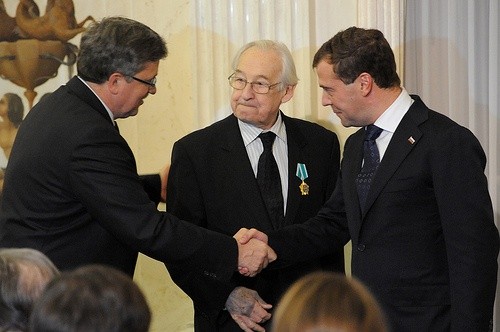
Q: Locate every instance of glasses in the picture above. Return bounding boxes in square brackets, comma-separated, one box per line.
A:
[109, 71, 158, 90]
[227, 72, 282, 94]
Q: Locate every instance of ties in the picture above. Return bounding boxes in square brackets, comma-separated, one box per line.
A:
[356, 124, 382, 218]
[257, 132, 285, 231]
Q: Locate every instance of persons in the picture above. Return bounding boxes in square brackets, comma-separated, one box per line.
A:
[0, 17, 387, 332]
[239, 26, 498, 332]
